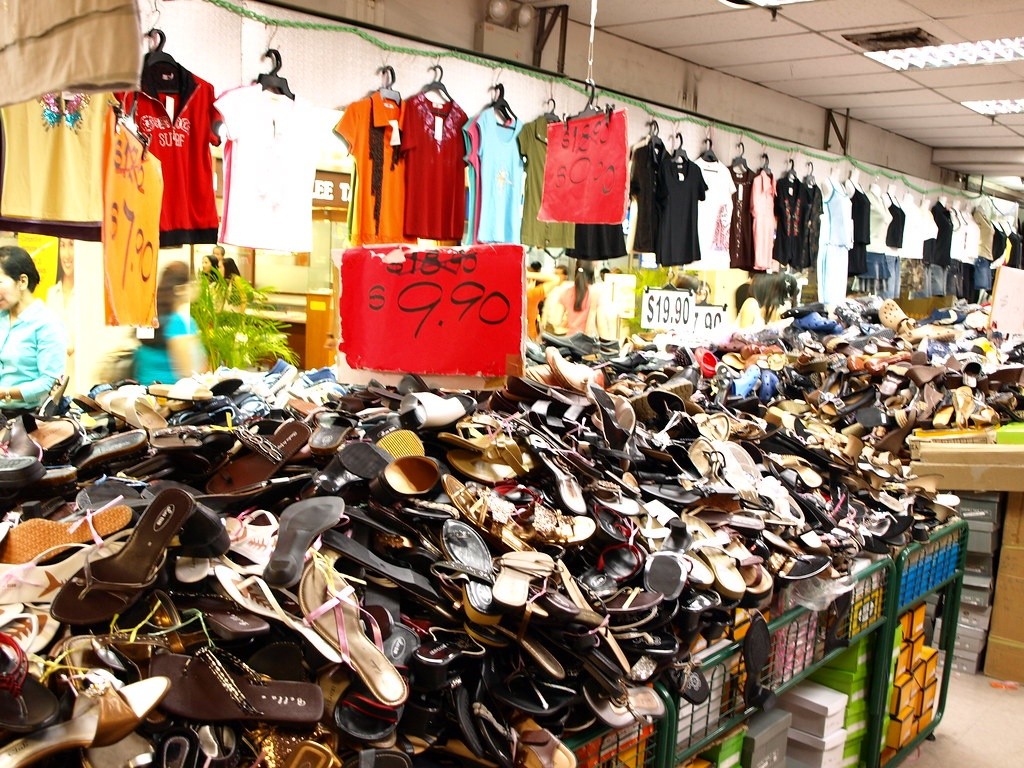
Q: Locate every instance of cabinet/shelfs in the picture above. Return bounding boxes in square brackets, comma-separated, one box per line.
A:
[652, 558, 897, 768]
[872, 520, 970, 768]
[565, 699, 669, 768]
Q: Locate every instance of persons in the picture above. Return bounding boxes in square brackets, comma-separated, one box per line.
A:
[530, 260, 546, 318]
[736, 268, 866, 319]
[526, 267, 561, 344]
[736, 272, 775, 337]
[0, 245, 66, 410]
[48, 238, 74, 357]
[554, 259, 600, 338]
[817, 165, 1024, 304]
[675, 274, 701, 304]
[553, 264, 570, 286]
[132, 261, 206, 388]
[212, 246, 240, 279]
[600, 269, 610, 282]
[202, 255, 219, 283]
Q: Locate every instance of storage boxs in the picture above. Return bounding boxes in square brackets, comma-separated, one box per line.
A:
[566, 422, 1024, 768]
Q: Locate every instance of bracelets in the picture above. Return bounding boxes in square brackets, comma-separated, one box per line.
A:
[4, 388, 11, 404]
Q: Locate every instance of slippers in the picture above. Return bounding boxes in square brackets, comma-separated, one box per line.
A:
[0, 299, 1024, 768]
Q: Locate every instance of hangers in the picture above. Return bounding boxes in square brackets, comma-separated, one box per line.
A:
[258, 49, 295, 99]
[379, 66, 402, 106]
[648, 121, 662, 147]
[807, 162, 817, 185]
[492, 83, 518, 131]
[143, 30, 184, 93]
[762, 154, 771, 174]
[702, 138, 718, 162]
[732, 143, 748, 171]
[563, 83, 616, 130]
[787, 159, 798, 180]
[422, 65, 453, 116]
[114, 94, 153, 160]
[673, 133, 688, 163]
[545, 99, 560, 124]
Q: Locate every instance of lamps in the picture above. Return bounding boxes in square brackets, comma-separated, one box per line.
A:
[475, 0, 534, 65]
